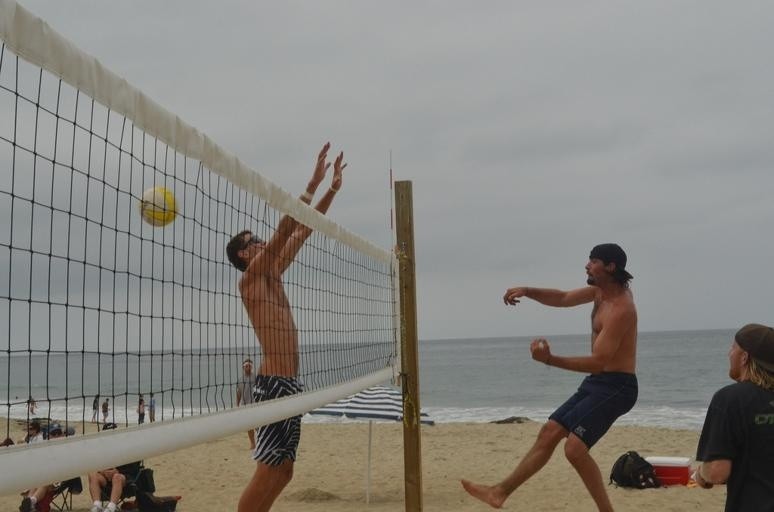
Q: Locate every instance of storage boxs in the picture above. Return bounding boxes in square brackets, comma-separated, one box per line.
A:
[641, 451, 695, 487]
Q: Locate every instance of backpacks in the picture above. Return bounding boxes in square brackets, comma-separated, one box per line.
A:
[609, 450, 661, 489]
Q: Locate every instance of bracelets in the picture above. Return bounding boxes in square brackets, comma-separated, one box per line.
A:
[327, 184, 338, 194]
[299, 188, 314, 201]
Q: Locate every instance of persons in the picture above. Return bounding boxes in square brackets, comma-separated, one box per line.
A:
[690, 320, 773, 512]
[225, 140, 351, 512]
[234, 358, 258, 450]
[453, 241, 640, 510]
[0, 391, 156, 511]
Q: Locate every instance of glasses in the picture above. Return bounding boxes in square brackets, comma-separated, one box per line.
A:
[242, 234, 263, 251]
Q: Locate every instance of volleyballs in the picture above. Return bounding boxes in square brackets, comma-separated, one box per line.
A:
[141, 188, 177, 226]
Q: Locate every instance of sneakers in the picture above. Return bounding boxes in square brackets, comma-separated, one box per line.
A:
[20, 497, 37, 512]
[90, 505, 115, 512]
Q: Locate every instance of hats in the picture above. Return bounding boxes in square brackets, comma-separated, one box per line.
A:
[102, 423, 118, 430]
[46, 423, 63, 434]
[735, 323, 774, 376]
[588, 243, 634, 280]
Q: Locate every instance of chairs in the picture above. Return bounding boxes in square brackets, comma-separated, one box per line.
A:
[97, 457, 161, 512]
[46, 481, 79, 511]
